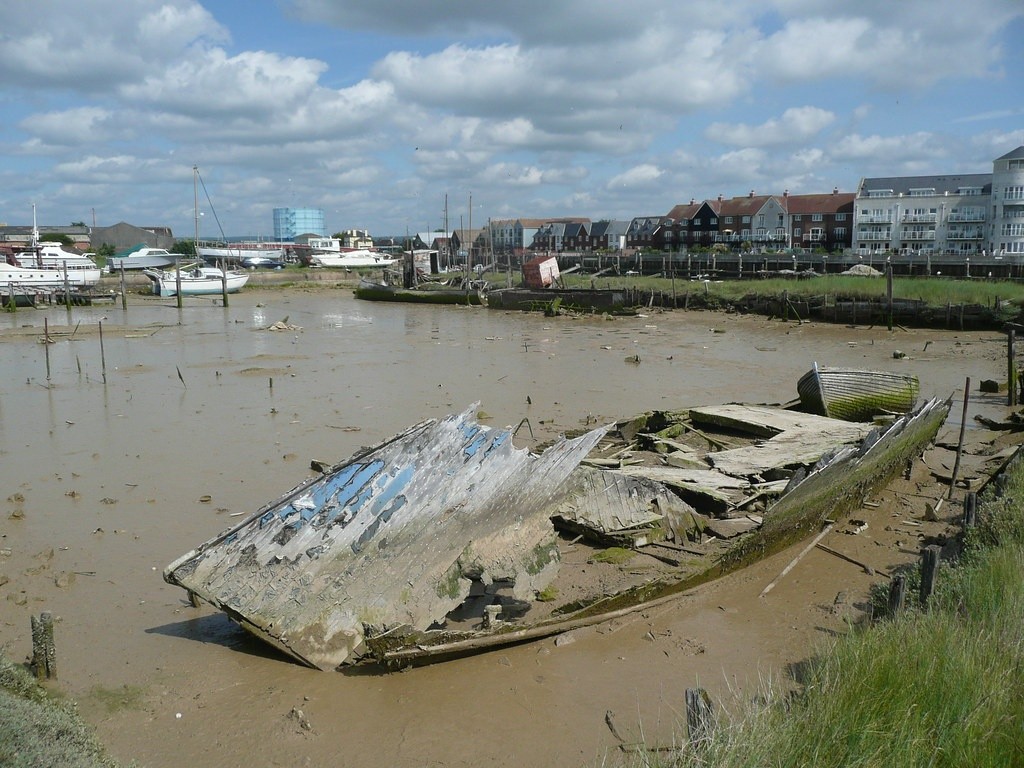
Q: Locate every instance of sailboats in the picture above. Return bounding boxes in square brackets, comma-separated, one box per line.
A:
[143, 164, 250, 297]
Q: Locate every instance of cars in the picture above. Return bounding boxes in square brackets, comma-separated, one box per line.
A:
[240, 256, 287, 271]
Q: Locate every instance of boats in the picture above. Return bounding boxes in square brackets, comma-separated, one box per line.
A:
[106, 246, 186, 271]
[194, 245, 288, 268]
[0, 204, 119, 307]
[797, 361, 920, 421]
[354, 269, 481, 304]
[487, 288, 643, 311]
[305, 248, 401, 269]
